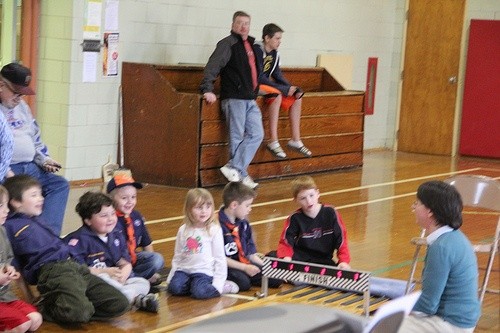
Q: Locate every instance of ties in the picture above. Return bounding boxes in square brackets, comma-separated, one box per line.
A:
[224, 221, 251, 264]
[117, 213, 137, 265]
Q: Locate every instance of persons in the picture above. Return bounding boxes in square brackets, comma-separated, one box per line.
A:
[216, 181, 288, 291]
[253, 23, 313, 159]
[106, 174, 164, 286]
[363, 182, 480, 333]
[199, 11, 264, 190]
[0, 186, 42, 333]
[165, 187, 239, 300]
[64, 191, 159, 313]
[277, 175, 351, 270]
[0, 61, 69, 236]
[1, 174, 130, 323]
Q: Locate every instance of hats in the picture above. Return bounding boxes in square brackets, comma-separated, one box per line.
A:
[106, 175, 143, 189]
[1, 62, 36, 96]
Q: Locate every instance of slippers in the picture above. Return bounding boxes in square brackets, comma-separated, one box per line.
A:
[264, 141, 286, 159]
[286, 142, 313, 158]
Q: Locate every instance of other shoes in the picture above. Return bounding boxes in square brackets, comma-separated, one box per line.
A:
[221, 163, 240, 183]
[224, 280, 239, 296]
[150, 273, 163, 286]
[133, 294, 158, 312]
[242, 173, 259, 190]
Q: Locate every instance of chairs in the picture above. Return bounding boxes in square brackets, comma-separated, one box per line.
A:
[405, 175, 500, 303]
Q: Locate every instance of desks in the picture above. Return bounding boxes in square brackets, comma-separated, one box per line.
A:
[122, 60, 367, 188]
